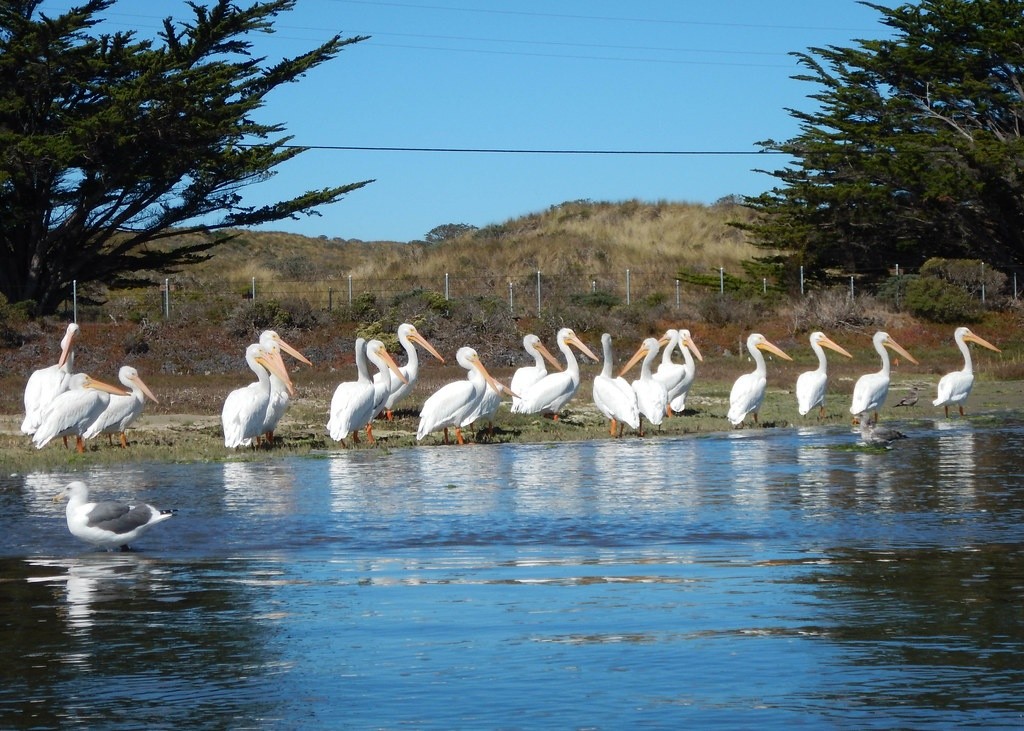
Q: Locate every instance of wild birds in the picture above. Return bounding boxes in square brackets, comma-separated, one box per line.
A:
[325, 321, 445, 452]
[849, 331, 919, 424]
[796, 331, 853, 419]
[55, 481, 179, 552]
[416, 345, 521, 446]
[931, 326, 1002, 418]
[592, 333, 641, 438]
[221, 330, 313, 452]
[727, 333, 794, 428]
[618, 327, 703, 438]
[511, 327, 599, 423]
[21, 322, 159, 454]
